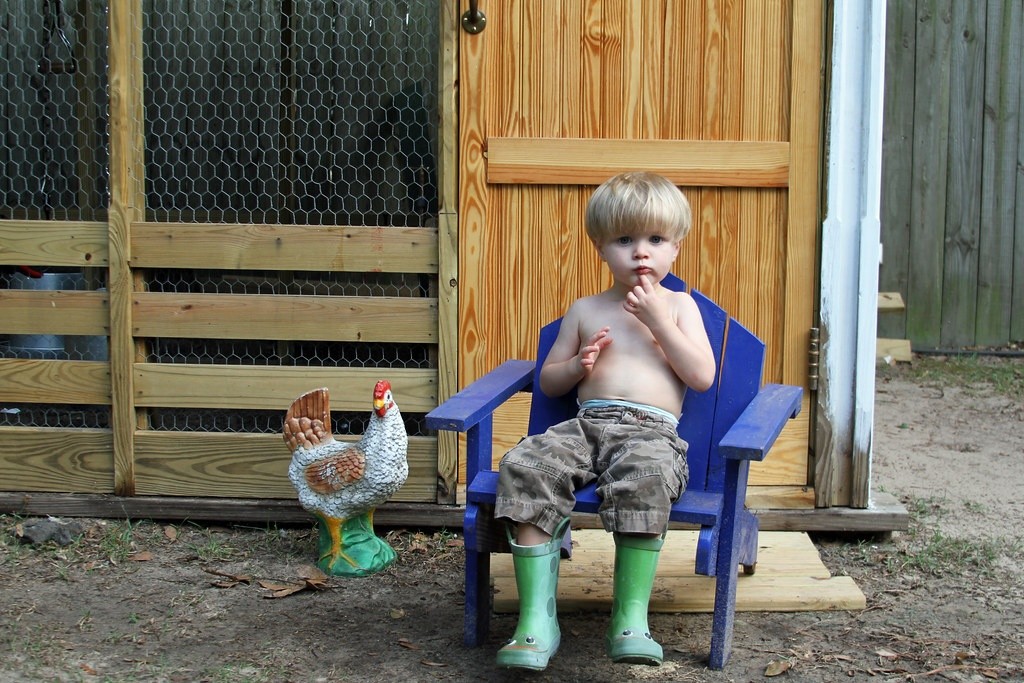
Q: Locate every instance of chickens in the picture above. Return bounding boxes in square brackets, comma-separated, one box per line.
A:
[283, 380, 410, 571]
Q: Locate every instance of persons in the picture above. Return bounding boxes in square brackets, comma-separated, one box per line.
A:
[493, 172, 716, 671]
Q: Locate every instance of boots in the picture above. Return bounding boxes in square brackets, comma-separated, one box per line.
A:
[605, 523, 669, 665]
[497, 517, 571, 670]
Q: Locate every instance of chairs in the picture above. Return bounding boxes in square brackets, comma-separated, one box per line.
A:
[426, 268, 806, 671]
